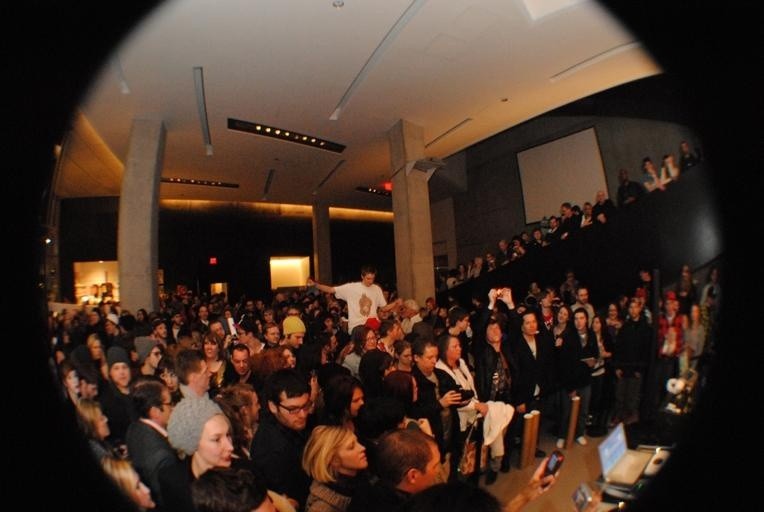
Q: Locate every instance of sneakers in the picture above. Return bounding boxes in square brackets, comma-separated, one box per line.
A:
[556, 438, 566, 449]
[576, 435, 588, 446]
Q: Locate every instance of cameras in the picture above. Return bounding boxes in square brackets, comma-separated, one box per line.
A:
[451, 383, 474, 401]
[231, 334, 239, 341]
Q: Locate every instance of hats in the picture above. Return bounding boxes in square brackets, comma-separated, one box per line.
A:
[166, 397, 224, 457]
[282, 315, 307, 337]
[134, 336, 161, 366]
[106, 346, 132, 367]
[106, 314, 119, 326]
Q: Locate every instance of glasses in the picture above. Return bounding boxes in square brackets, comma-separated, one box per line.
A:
[278, 398, 313, 415]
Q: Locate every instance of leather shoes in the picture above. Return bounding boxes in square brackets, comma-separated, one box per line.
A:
[485, 470, 498, 486]
[500, 458, 510, 473]
[535, 448, 546, 458]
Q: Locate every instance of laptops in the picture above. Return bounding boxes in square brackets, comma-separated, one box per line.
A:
[597, 422, 653, 486]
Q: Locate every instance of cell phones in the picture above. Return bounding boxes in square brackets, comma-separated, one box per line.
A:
[541, 450, 564, 489]
